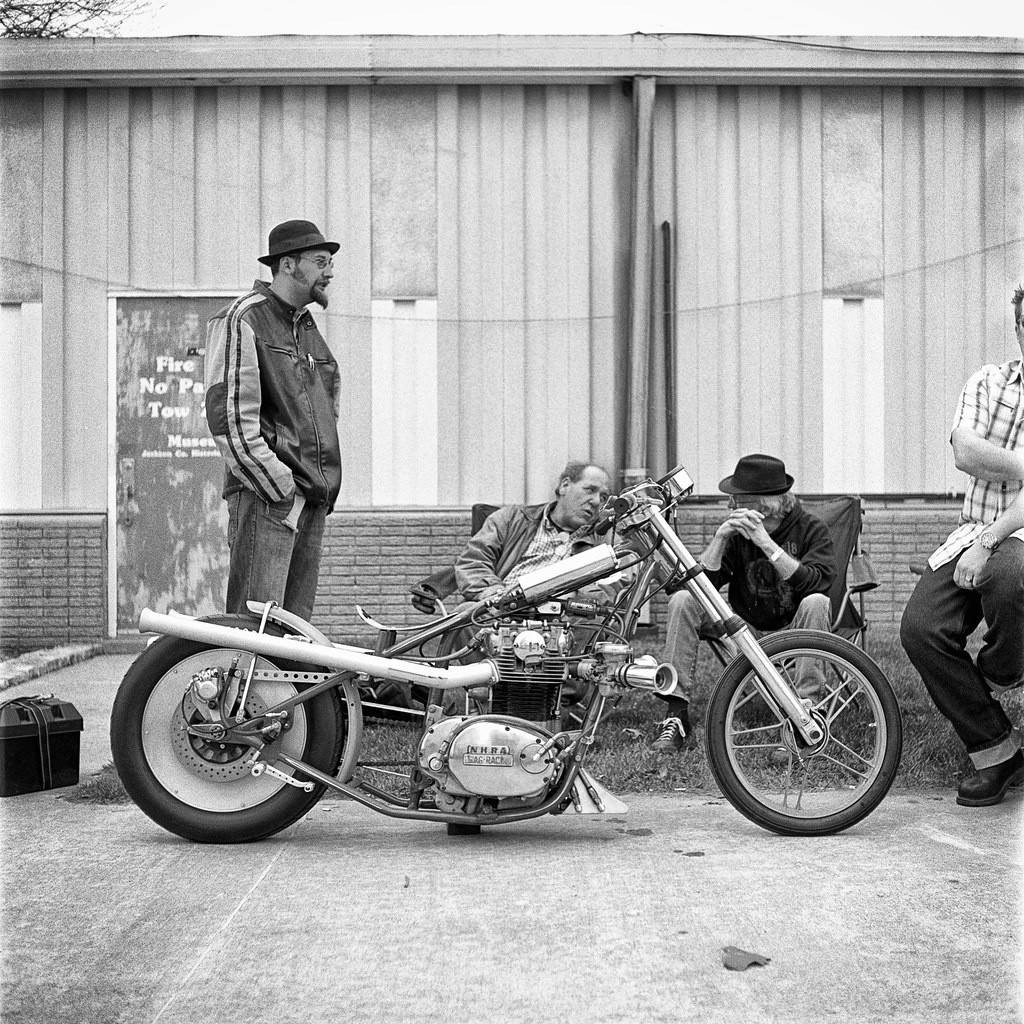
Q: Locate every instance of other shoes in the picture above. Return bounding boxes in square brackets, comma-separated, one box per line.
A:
[957, 748, 1024, 804]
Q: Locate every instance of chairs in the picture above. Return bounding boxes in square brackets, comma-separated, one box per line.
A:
[664, 495, 882, 714]
[408, 504, 639, 724]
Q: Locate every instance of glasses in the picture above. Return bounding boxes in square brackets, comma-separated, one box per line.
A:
[556, 529, 570, 561]
[293, 252, 335, 268]
[729, 494, 758, 505]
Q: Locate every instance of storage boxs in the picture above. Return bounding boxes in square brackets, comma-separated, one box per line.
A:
[0, 696, 84, 798]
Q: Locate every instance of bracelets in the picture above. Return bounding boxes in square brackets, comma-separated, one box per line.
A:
[768, 547, 785, 563]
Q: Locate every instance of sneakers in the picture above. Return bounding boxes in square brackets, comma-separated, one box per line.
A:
[648, 712, 692, 752]
[773, 745, 798, 763]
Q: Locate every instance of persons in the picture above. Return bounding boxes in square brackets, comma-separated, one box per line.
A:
[900, 286, 1024, 806]
[650, 454, 838, 759]
[450, 461, 631, 720]
[204, 219, 343, 636]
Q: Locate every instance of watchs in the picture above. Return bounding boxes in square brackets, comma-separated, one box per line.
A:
[978, 530, 999, 550]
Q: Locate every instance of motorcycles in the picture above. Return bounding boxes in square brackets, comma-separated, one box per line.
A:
[105, 470, 906, 843]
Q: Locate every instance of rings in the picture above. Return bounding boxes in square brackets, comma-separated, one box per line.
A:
[965, 578, 972, 582]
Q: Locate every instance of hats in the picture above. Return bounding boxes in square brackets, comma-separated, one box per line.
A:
[255, 220, 340, 262]
[718, 453, 794, 497]
